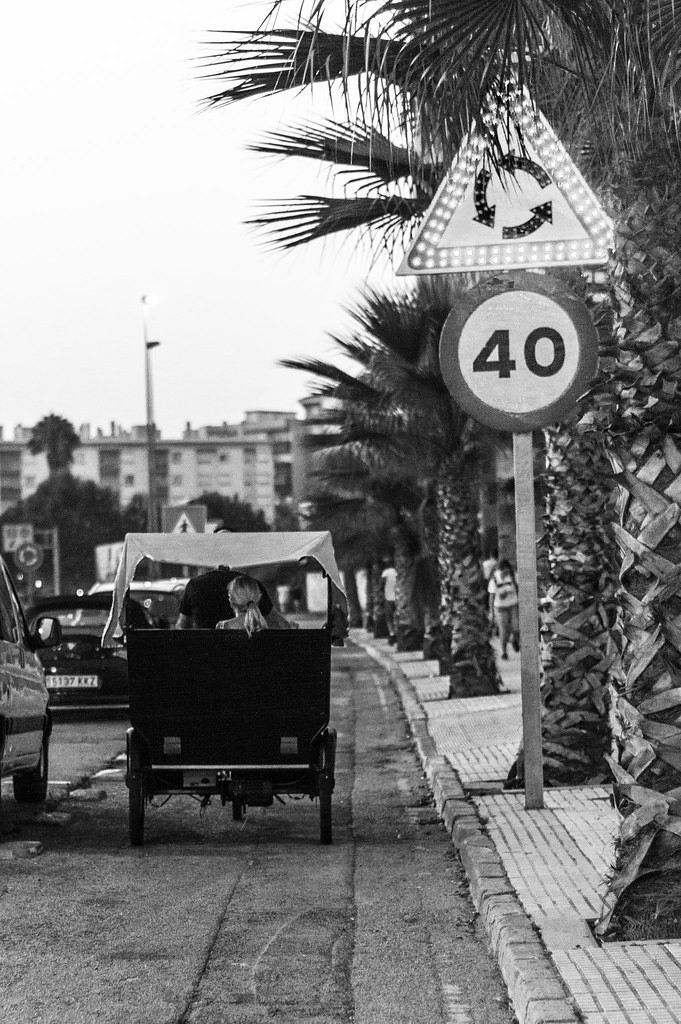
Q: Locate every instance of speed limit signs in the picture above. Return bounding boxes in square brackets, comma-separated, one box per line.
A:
[442, 271, 600, 435]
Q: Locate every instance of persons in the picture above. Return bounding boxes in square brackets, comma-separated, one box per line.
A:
[482, 549, 520, 659]
[215, 575, 288, 639]
[380, 559, 399, 646]
[175, 530, 291, 630]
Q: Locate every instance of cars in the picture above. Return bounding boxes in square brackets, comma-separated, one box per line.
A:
[0, 553, 63, 805]
[18, 591, 166, 719]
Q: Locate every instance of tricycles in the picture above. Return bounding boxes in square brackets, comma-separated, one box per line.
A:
[119, 532, 339, 849]
